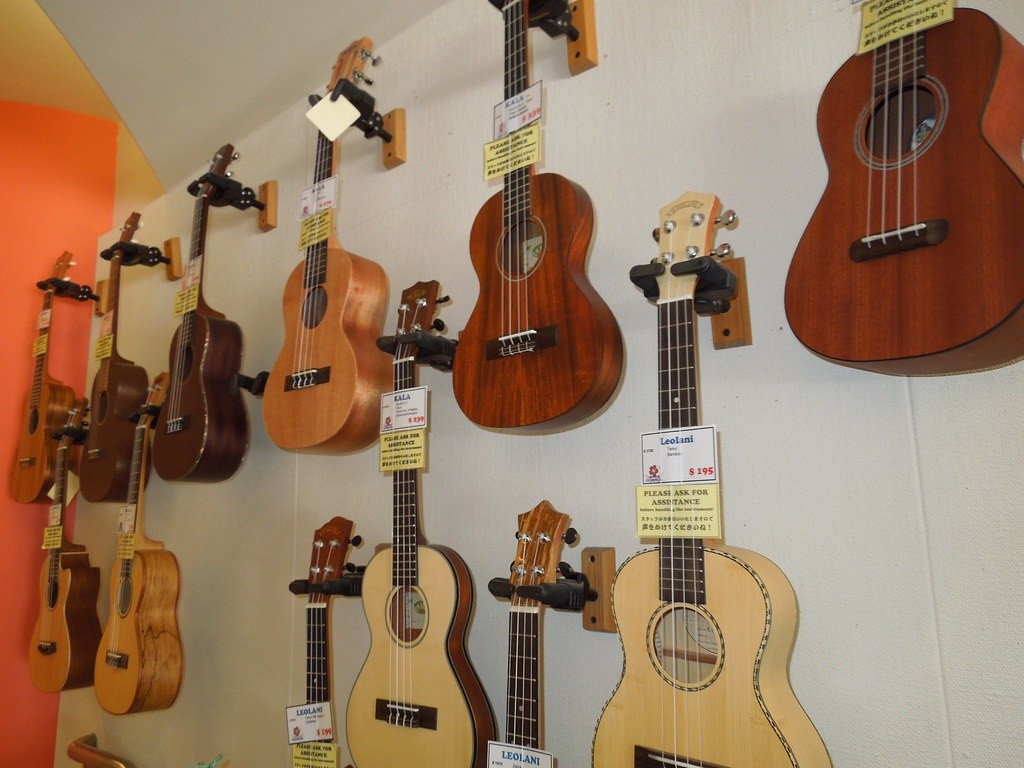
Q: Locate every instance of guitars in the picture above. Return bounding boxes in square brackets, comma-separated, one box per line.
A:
[25, 396, 102, 695]
[587, 187, 836, 768]
[7, 251, 76, 505]
[93, 370, 186, 717]
[261, 36, 395, 459]
[286, 515, 363, 768]
[78, 212, 151, 504]
[150, 142, 251, 485]
[486, 497, 579, 768]
[783, 0, 1024, 378]
[341, 274, 502, 768]
[448, 0, 629, 437]
